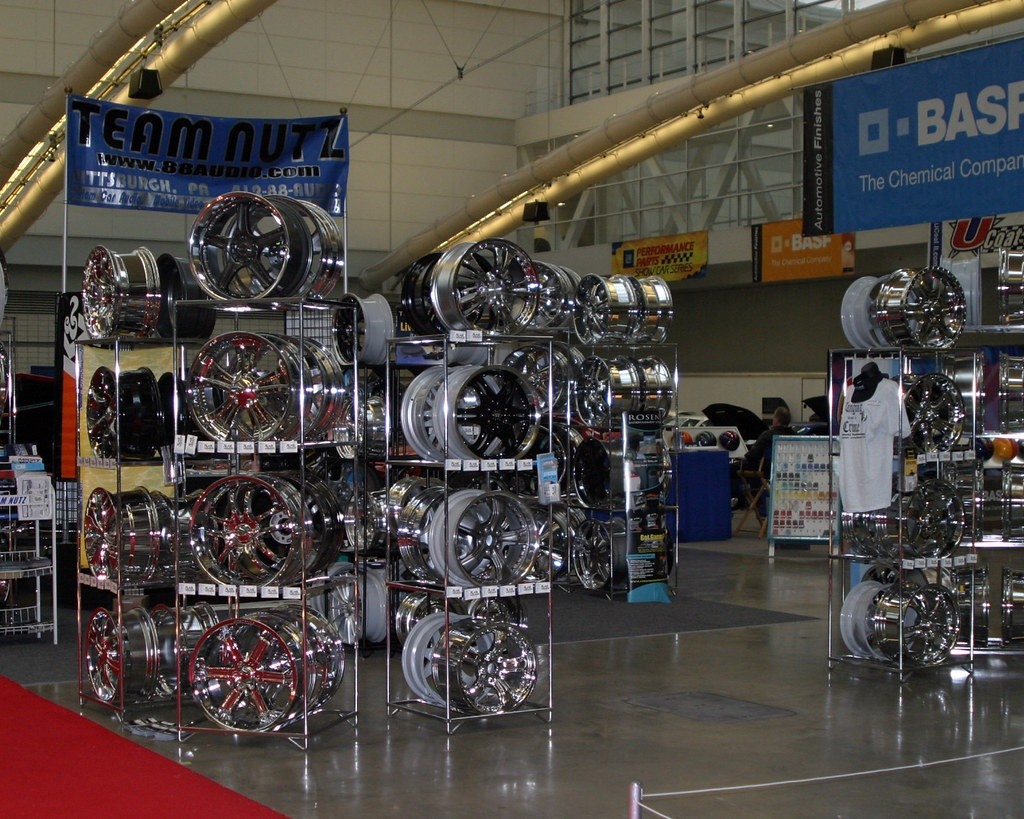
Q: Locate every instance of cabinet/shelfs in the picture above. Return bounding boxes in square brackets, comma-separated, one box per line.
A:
[78, 298, 1023, 751]
[0, 315, 60, 646]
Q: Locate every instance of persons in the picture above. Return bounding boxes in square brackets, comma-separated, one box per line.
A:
[750, 407, 810, 549]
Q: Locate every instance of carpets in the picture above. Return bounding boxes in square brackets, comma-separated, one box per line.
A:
[0, 674, 292, 819]
